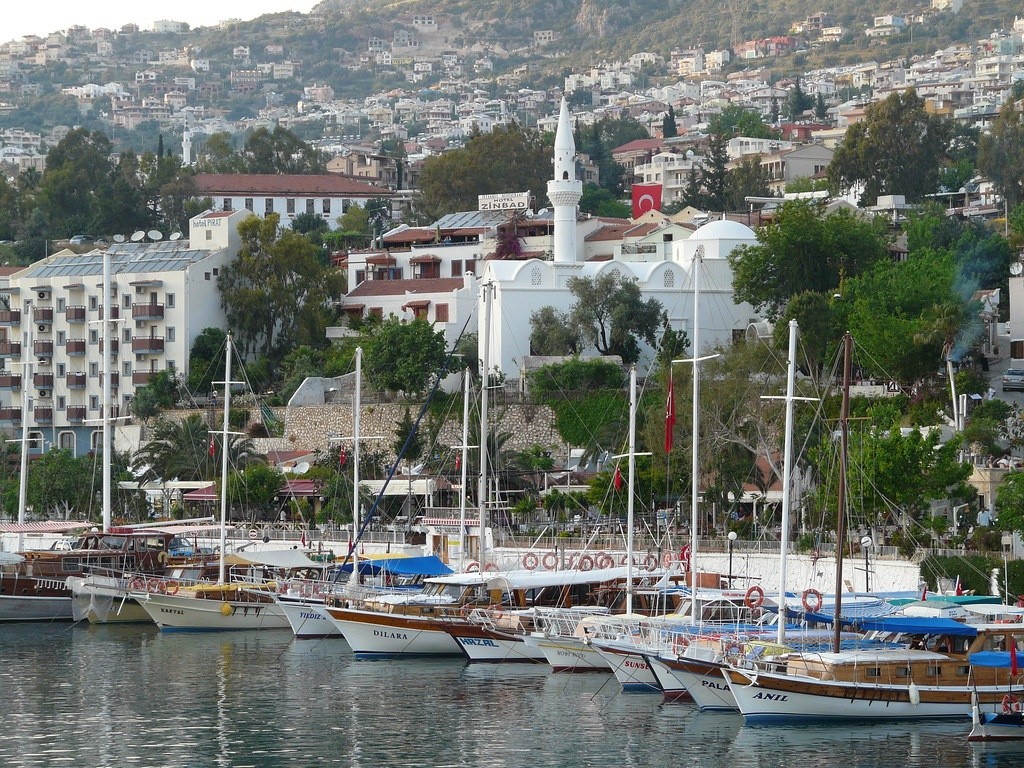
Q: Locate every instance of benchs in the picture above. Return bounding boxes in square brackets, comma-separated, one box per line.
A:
[190, 396, 225, 408]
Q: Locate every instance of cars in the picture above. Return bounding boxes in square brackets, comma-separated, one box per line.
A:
[1003, 367, 1024, 392]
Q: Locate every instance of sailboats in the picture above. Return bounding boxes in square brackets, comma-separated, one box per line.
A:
[0, 252, 410, 624]
[719, 330, 1024, 726]
[230, 244, 1024, 712]
[76, 327, 346, 632]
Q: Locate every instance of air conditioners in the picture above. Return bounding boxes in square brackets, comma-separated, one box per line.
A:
[472, 252, 482, 259]
[38, 292, 50, 300]
[136, 286, 144, 294]
[136, 320, 146, 328]
[38, 389, 51, 399]
[39, 357, 50, 366]
[38, 324, 50, 333]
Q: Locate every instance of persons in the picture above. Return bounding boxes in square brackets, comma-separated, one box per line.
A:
[280, 510, 288, 521]
[976, 505, 994, 528]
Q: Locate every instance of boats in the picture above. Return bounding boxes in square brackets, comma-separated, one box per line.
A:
[968, 650, 1024, 741]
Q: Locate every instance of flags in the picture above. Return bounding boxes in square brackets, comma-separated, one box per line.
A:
[1010, 635, 1019, 677]
[630, 182, 664, 221]
[208, 441, 215, 456]
[955, 576, 962, 595]
[301, 529, 306, 547]
[664, 373, 677, 454]
[681, 543, 692, 571]
[455, 453, 460, 472]
[348, 534, 352, 551]
[615, 465, 622, 492]
[340, 448, 347, 465]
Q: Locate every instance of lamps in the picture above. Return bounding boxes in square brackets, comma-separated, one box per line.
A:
[137, 354, 144, 361]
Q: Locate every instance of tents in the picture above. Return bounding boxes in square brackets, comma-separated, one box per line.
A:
[183, 477, 325, 500]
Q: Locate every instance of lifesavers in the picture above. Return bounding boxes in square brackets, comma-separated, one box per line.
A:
[673, 637, 688, 655]
[523, 552, 538, 570]
[802, 588, 823, 611]
[488, 604, 503, 621]
[466, 562, 479, 572]
[300, 583, 330, 598]
[1002, 694, 1020, 712]
[745, 585, 764, 608]
[621, 554, 635, 565]
[594, 552, 609, 569]
[569, 553, 593, 572]
[542, 552, 558, 570]
[996, 620, 1014, 623]
[484, 562, 499, 572]
[128, 578, 179, 595]
[643, 555, 657, 572]
[725, 643, 744, 665]
[600, 555, 614, 569]
[461, 605, 473, 620]
[157, 551, 168, 563]
[663, 551, 680, 569]
